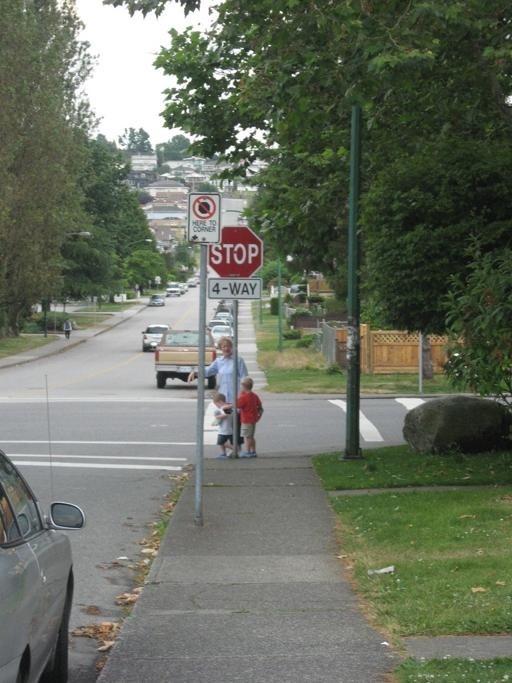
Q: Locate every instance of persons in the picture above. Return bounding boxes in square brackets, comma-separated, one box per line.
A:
[187, 338, 263, 459]
[64, 318, 72, 340]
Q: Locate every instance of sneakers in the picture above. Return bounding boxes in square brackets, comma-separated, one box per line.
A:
[217, 449, 256, 458]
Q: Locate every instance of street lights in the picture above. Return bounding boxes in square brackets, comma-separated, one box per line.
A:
[220, 208, 285, 350]
[123, 238, 152, 250]
[43, 232, 90, 338]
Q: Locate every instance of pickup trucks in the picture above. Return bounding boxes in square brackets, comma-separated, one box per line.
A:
[156, 329, 216, 389]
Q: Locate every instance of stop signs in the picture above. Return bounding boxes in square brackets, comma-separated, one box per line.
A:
[208, 227, 263, 278]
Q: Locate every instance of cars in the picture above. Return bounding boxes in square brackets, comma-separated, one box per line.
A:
[205, 298, 238, 349]
[142, 324, 172, 352]
[0, 449, 85, 683]
[148, 268, 200, 306]
